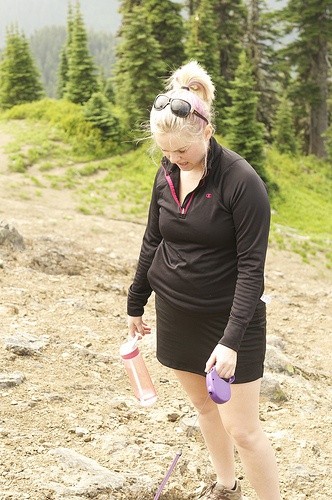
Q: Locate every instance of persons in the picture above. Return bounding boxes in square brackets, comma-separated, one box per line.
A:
[125, 61, 282, 500]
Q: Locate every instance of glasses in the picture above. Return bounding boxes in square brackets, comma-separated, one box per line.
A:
[153, 94, 208, 125]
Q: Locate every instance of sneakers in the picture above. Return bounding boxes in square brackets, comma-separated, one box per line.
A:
[191, 477, 242, 500]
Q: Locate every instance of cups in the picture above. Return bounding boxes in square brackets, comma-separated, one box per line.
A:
[119, 332, 157, 407]
